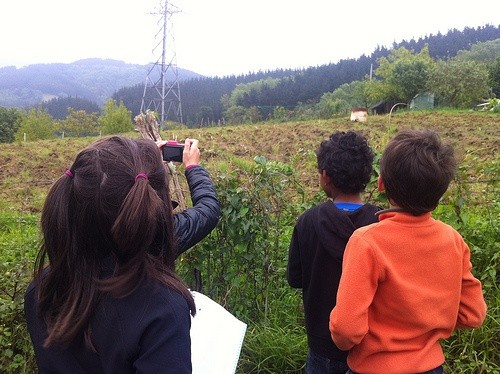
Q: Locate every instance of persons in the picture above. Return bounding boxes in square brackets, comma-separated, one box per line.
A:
[286, 132, 384, 374]
[329, 131, 487, 374]
[24, 137, 196, 374]
[141, 138, 220, 276]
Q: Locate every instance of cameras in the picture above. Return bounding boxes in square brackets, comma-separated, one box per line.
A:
[162, 141, 184, 162]
